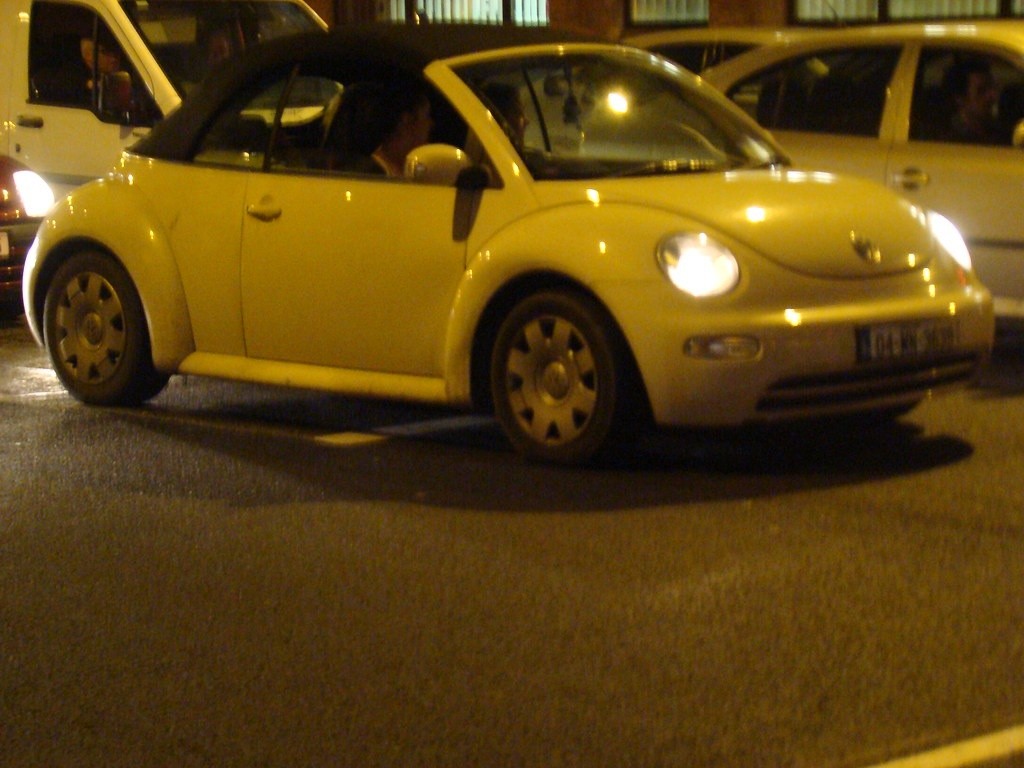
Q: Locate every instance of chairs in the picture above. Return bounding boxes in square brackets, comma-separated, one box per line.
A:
[309, 81, 392, 174]
[424, 94, 467, 150]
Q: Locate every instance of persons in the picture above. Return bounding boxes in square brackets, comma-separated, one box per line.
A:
[922, 57, 1012, 145]
[190, 19, 233, 67]
[483, 79, 651, 178]
[349, 85, 433, 179]
[46, 33, 121, 117]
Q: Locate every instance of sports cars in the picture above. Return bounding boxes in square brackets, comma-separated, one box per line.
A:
[20, 19, 997, 472]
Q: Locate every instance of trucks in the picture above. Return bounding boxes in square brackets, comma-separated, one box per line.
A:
[0, 0, 350, 213]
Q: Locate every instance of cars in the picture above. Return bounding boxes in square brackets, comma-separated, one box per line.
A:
[0, 154, 59, 317]
[571, 15, 1024, 342]
[599, 27, 826, 110]
